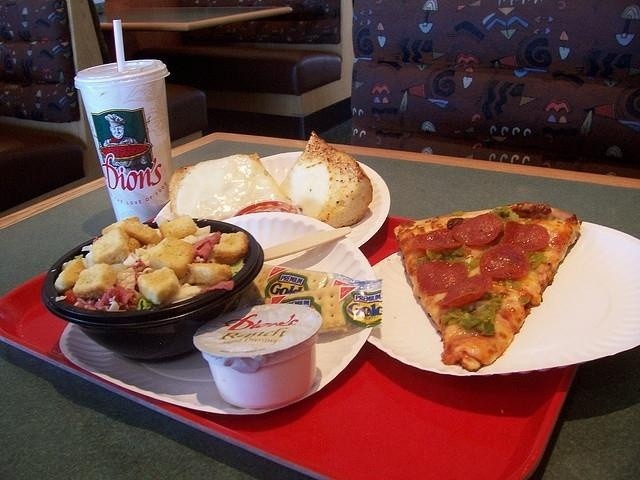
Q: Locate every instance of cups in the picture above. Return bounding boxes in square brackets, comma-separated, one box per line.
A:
[73, 60, 175, 225]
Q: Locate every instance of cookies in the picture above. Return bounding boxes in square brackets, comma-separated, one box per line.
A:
[246, 263, 350, 335]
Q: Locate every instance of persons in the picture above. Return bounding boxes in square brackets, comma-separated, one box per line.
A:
[98, 114, 148, 168]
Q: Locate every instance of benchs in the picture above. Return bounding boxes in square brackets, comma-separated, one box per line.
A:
[1, 1, 640, 479]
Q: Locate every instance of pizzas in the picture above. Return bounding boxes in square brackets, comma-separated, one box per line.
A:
[393, 201, 584, 372]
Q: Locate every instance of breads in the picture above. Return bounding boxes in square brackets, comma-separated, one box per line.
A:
[168, 153, 299, 221]
[279, 130, 373, 228]
[54, 215, 250, 306]
